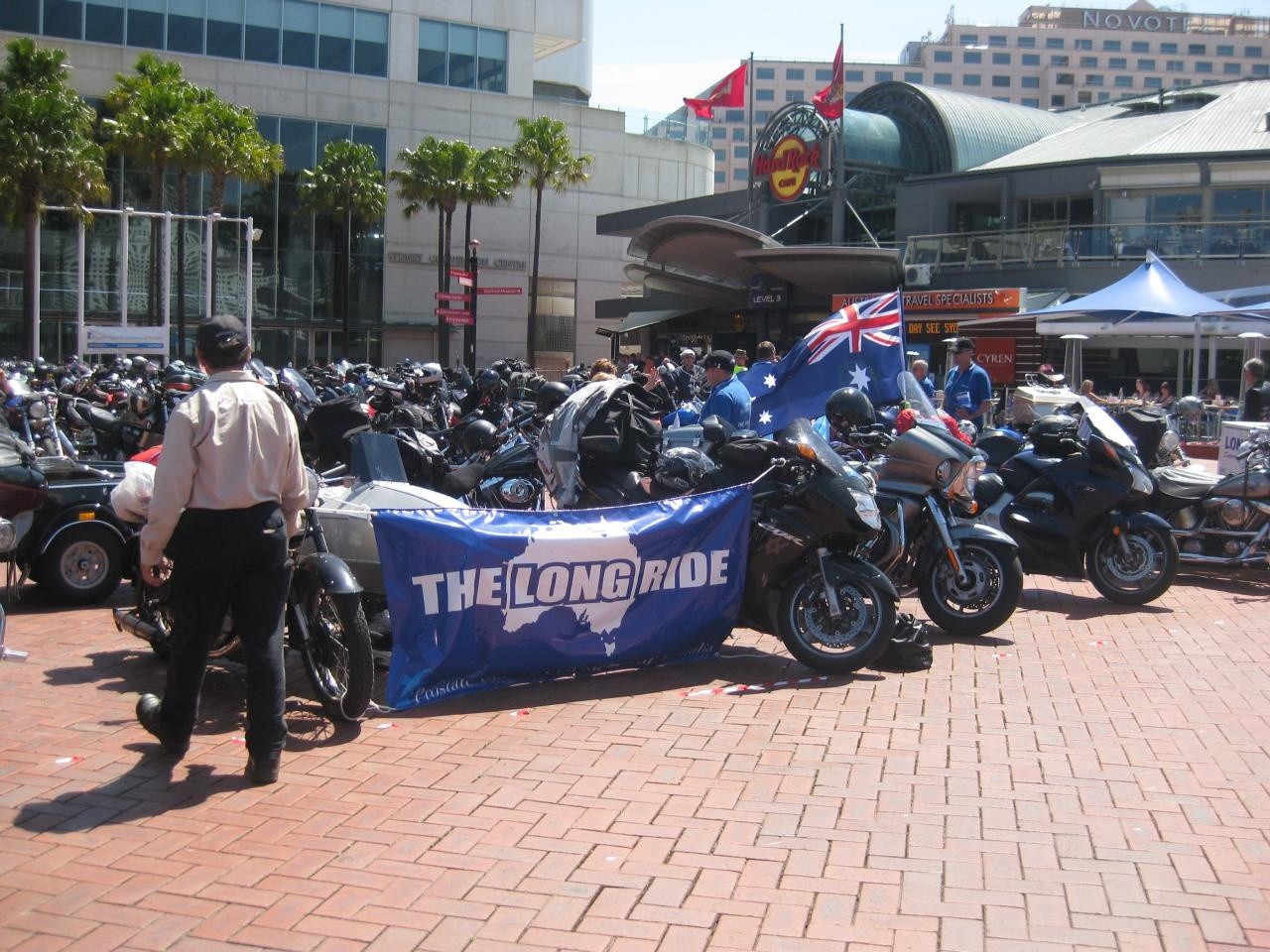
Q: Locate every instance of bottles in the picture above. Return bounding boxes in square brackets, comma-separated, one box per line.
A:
[1119, 388, 1123, 399]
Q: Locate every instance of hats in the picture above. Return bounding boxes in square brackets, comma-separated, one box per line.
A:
[948, 338, 975, 353]
[696, 350, 735, 371]
[734, 349, 749, 360]
[196, 315, 247, 349]
[625, 364, 638, 373]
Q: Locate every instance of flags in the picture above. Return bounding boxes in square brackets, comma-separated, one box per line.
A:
[740, 284, 907, 436]
[811, 41, 844, 120]
[682, 63, 746, 121]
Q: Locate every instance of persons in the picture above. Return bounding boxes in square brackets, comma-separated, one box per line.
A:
[1197, 378, 1221, 400]
[1150, 381, 1176, 414]
[1132, 378, 1151, 400]
[1237, 358, 1270, 423]
[910, 358, 937, 409]
[943, 339, 992, 434]
[136, 314, 312, 783]
[589, 337, 781, 401]
[1080, 379, 1103, 404]
[697, 351, 751, 430]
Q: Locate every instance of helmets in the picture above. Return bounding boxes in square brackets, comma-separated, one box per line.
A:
[122, 358, 133, 371]
[479, 370, 500, 392]
[133, 356, 149, 368]
[35, 357, 45, 365]
[957, 420, 979, 443]
[538, 382, 572, 414]
[654, 447, 707, 491]
[664, 402, 700, 427]
[74, 377, 96, 396]
[418, 363, 443, 384]
[491, 360, 512, 379]
[826, 387, 876, 431]
[463, 420, 498, 456]
[130, 382, 155, 416]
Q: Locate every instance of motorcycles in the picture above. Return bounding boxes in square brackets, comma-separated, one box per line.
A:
[0, 349, 1269, 725]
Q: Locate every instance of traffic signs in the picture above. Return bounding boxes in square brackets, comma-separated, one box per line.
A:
[476, 286, 523, 296]
[458, 277, 472, 286]
[433, 308, 471, 317]
[435, 292, 472, 302]
[443, 315, 473, 326]
[447, 269, 470, 277]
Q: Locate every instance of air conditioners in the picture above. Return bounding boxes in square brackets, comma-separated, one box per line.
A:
[905, 263, 931, 287]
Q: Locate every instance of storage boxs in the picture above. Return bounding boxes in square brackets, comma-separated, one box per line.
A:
[663, 424, 715, 453]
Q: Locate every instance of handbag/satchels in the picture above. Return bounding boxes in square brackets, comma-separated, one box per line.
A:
[578, 384, 663, 472]
[306, 393, 374, 465]
[867, 610, 933, 669]
[717, 438, 799, 485]
[111, 462, 186, 521]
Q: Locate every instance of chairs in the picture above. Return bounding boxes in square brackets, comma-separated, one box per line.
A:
[1108, 394, 1246, 440]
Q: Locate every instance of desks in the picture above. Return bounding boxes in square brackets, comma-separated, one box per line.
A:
[1105, 400, 1141, 406]
[1202, 405, 1237, 438]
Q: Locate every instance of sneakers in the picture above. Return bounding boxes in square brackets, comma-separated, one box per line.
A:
[136, 693, 189, 754]
[245, 748, 281, 782]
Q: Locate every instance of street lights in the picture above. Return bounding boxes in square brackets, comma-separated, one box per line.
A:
[466, 239, 481, 375]
